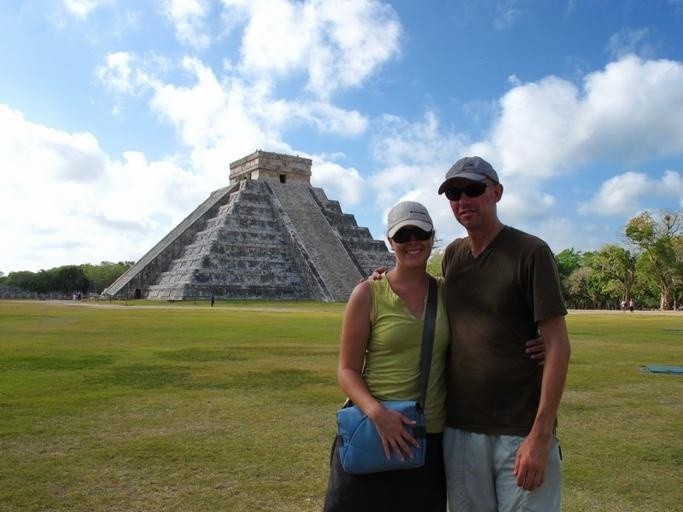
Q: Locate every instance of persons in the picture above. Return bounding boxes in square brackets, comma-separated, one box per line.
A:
[620, 298, 634, 313]
[322, 201, 553, 511]
[358, 156, 572, 512]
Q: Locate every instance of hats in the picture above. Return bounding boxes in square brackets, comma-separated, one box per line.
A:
[387, 200, 433, 238]
[438, 155, 499, 195]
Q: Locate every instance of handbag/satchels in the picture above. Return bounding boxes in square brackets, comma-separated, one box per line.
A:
[337, 400, 427, 474]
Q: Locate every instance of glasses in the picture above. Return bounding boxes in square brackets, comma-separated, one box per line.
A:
[446, 183, 486, 201]
[392, 227, 432, 244]
